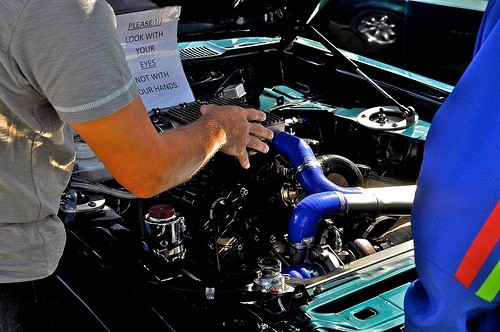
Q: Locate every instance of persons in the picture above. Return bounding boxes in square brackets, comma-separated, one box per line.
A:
[1, 0, 277, 330]
